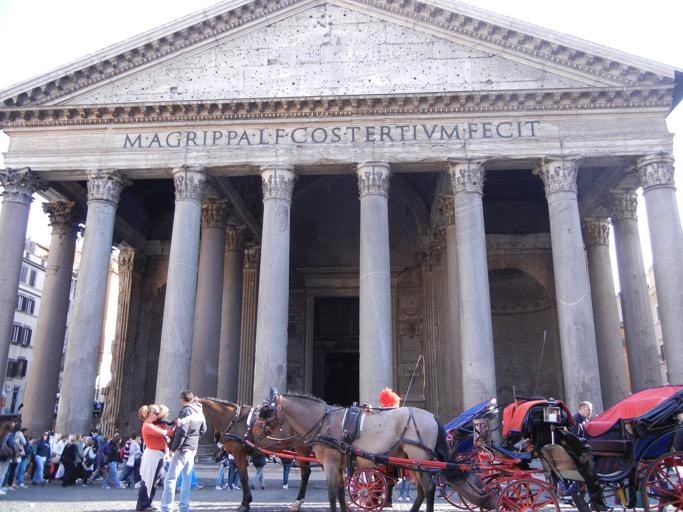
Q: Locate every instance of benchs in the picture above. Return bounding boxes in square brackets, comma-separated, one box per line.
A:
[584, 438, 631, 456]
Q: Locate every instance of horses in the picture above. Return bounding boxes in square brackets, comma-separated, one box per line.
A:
[250, 385, 470, 512]
[193, 396, 313, 512]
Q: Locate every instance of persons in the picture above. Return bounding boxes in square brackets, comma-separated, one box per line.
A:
[435, 473, 447, 498]
[396, 471, 412, 502]
[0, 391, 295, 511]
[569, 400, 592, 438]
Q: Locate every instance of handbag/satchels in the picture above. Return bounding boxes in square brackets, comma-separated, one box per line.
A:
[85, 454, 93, 467]
[55, 462, 65, 478]
[18, 443, 25, 456]
[0, 446, 13, 461]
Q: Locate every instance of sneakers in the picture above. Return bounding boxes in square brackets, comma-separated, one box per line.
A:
[250, 484, 264, 490]
[136, 506, 157, 512]
[283, 484, 288, 489]
[116, 481, 141, 488]
[0, 482, 28, 495]
[194, 485, 204, 490]
[216, 483, 240, 491]
